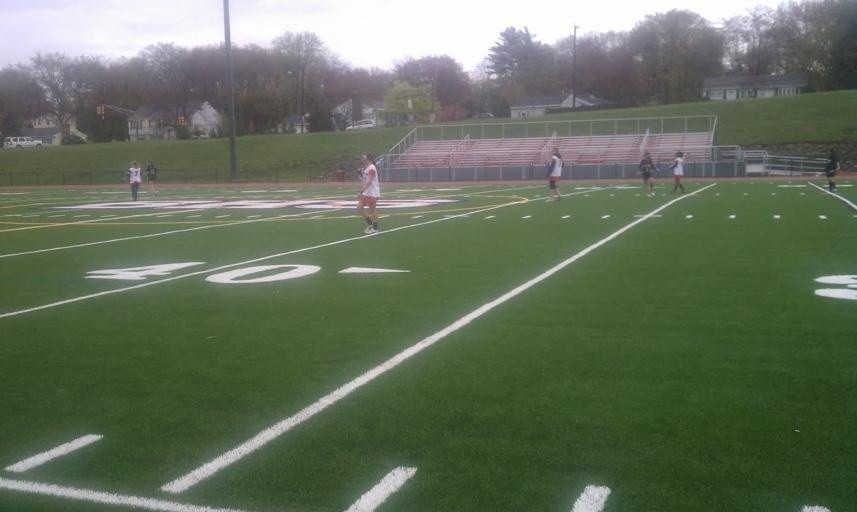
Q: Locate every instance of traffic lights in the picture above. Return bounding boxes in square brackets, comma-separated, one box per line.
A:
[97, 106, 105, 116]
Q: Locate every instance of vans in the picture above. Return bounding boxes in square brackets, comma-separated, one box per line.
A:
[3, 137, 42, 149]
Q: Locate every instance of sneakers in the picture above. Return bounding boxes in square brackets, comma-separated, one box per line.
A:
[546, 193, 562, 202]
[364, 224, 378, 233]
[668, 189, 687, 195]
[647, 192, 655, 198]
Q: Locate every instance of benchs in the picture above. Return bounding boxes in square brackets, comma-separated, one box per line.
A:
[392, 131, 710, 167]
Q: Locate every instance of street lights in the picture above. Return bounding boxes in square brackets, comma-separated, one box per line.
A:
[573, 24, 581, 111]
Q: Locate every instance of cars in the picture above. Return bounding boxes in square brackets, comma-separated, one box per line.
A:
[474, 112, 494, 118]
[61, 136, 87, 144]
[346, 119, 376, 131]
[190, 127, 209, 139]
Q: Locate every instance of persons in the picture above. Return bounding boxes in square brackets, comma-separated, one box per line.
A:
[638, 152, 659, 197]
[127, 161, 141, 202]
[823, 149, 840, 192]
[355, 152, 381, 236]
[144, 158, 160, 198]
[667, 151, 685, 194]
[544, 147, 562, 204]
